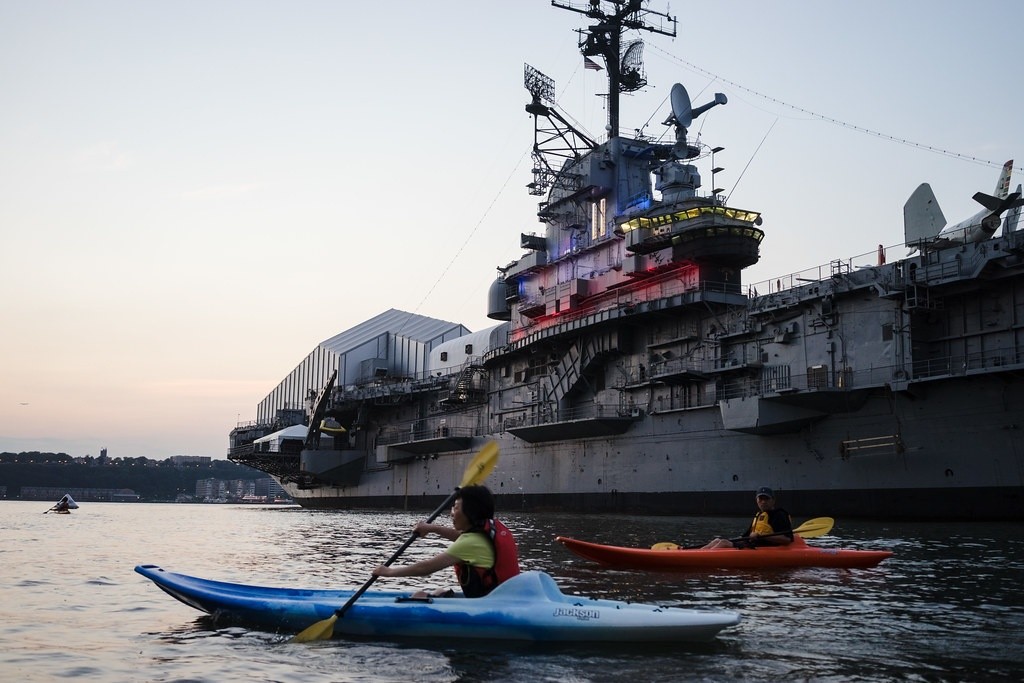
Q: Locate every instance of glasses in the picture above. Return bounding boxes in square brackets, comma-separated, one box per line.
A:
[451, 506, 463, 513]
[757, 497, 769, 503]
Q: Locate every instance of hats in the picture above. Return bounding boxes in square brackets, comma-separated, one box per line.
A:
[756, 486, 773, 498]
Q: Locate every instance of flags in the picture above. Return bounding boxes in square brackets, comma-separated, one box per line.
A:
[584, 56, 605, 72]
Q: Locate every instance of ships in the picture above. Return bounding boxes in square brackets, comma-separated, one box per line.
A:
[225, 1, 1024, 513]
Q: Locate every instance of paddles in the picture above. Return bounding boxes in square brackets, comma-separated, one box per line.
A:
[282, 439, 500, 643]
[43, 497, 68, 514]
[651, 515, 834, 554]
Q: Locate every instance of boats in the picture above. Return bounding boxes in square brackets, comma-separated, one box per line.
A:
[47, 510, 70, 514]
[556, 535, 896, 574]
[134, 563, 741, 644]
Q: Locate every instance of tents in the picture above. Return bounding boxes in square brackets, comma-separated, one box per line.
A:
[253, 424, 333, 453]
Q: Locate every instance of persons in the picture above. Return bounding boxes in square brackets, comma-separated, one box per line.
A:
[372, 486, 519, 598]
[628, 395, 635, 411]
[699, 487, 794, 550]
[877, 244, 885, 266]
[639, 363, 645, 379]
[50, 497, 70, 511]
